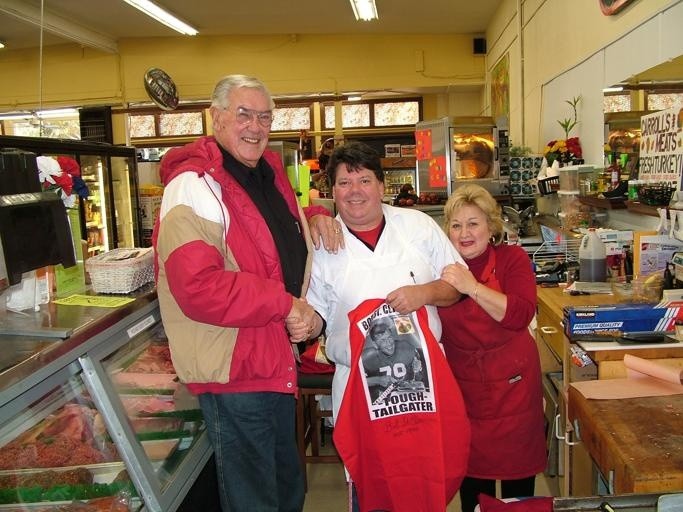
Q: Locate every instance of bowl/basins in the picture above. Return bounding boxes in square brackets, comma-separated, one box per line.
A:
[610, 275, 662, 306]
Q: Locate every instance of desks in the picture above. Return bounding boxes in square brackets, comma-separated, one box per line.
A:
[533, 282, 681, 497]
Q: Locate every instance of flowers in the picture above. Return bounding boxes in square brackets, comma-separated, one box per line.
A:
[542, 92, 584, 159]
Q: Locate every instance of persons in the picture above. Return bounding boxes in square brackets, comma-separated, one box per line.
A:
[286, 140, 469, 512]
[439, 183, 548, 510]
[149, 74, 344, 511]
[361, 321, 423, 406]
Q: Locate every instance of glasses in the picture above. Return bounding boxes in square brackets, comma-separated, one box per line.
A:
[225, 106, 275, 128]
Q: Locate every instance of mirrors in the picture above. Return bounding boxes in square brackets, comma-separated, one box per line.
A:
[535, 0, 683, 245]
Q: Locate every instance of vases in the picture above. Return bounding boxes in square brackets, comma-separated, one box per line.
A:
[543, 152, 563, 168]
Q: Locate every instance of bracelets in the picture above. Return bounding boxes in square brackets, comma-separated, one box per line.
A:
[472, 281, 483, 302]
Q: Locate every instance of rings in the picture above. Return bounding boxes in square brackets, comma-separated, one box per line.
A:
[334, 229, 340, 233]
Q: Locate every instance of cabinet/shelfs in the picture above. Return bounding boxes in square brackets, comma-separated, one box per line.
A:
[0, 284, 219, 510]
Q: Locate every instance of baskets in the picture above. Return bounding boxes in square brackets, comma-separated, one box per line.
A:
[636, 186, 677, 206]
[86, 247, 155, 296]
[538, 176, 560, 194]
[561, 213, 593, 230]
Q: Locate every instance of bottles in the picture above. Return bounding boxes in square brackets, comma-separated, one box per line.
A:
[579, 227, 606, 283]
[84, 198, 106, 257]
[384, 175, 413, 193]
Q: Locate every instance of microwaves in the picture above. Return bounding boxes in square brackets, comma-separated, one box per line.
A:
[448, 126, 507, 182]
